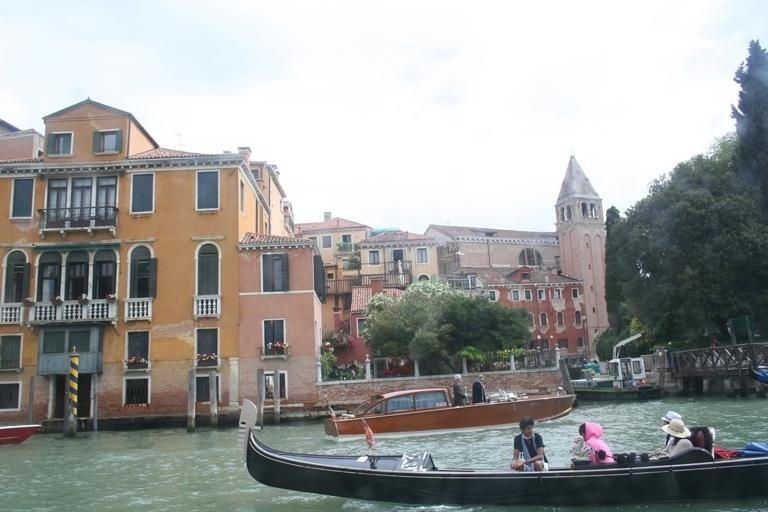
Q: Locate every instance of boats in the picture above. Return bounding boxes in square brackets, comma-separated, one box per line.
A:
[574, 386, 665, 400]
[321, 389, 577, 437]
[245, 427, 768, 505]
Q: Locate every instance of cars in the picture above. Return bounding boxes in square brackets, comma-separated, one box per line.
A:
[383, 364, 412, 377]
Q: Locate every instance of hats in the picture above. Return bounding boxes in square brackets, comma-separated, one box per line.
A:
[477, 374, 484, 380]
[661, 411, 684, 424]
[660, 419, 692, 439]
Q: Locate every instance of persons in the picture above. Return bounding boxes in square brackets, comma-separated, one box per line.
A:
[578, 422, 615, 464]
[453, 374, 469, 406]
[655, 418, 695, 460]
[511, 416, 549, 471]
[582, 359, 587, 365]
[660, 411, 681, 453]
[711, 336, 721, 366]
[472, 374, 487, 403]
